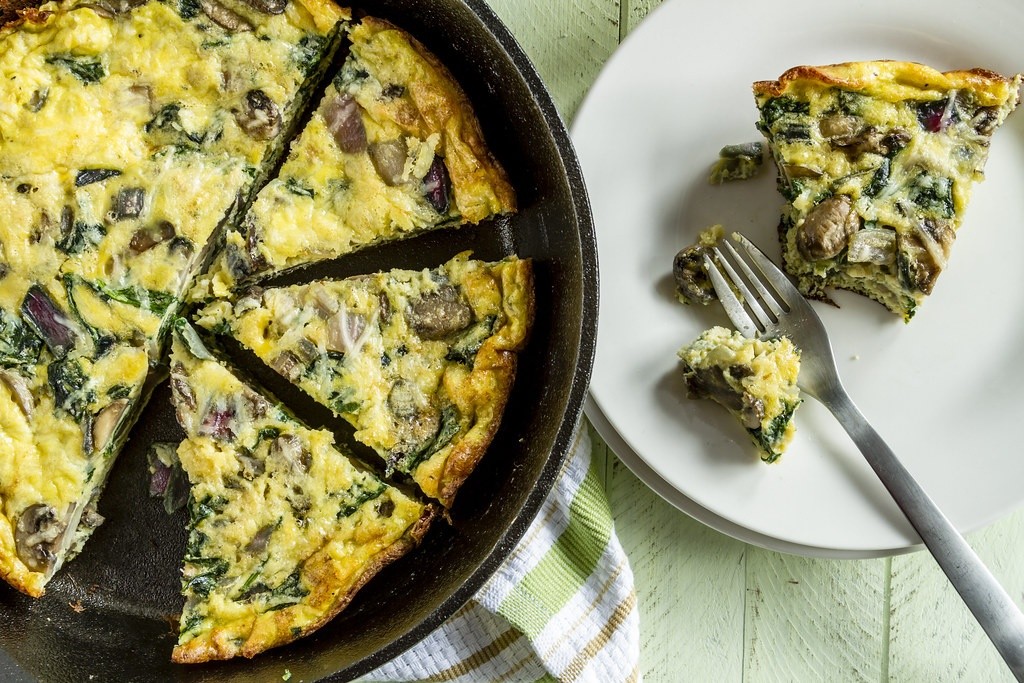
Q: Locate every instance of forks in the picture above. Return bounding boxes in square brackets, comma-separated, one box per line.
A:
[702, 233, 1023, 683]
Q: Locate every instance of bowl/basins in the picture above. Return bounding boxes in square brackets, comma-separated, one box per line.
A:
[0, 1, 602, 683]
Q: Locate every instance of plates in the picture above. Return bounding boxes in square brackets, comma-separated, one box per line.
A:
[553, 1, 1024, 560]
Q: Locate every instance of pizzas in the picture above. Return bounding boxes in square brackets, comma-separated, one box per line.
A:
[676, 58, 1024, 463]
[0, 0, 531, 665]
